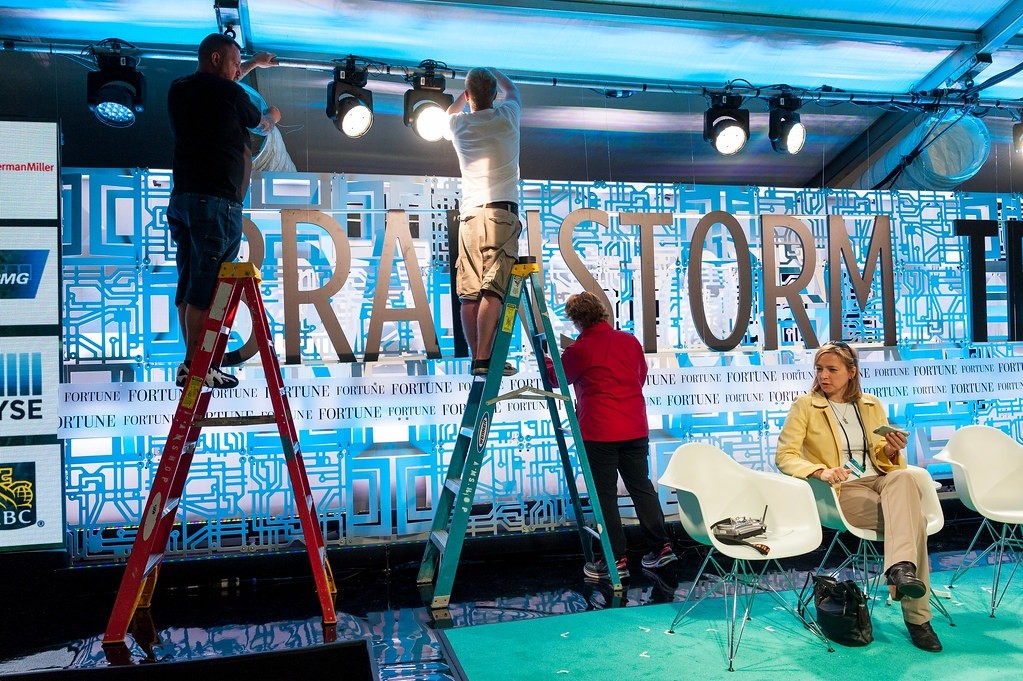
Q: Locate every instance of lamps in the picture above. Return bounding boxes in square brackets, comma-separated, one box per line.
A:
[403, 71, 455, 144]
[325, 69, 374, 141]
[702, 90, 751, 158]
[768, 93, 806, 155]
[82, 56, 148, 127]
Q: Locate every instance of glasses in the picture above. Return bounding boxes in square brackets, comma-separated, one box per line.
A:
[824, 341, 854, 360]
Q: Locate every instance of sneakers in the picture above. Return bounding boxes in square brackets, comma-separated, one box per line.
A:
[470, 357, 518, 376]
[643, 564, 679, 593]
[583, 552, 629, 579]
[584, 575, 629, 597]
[175, 359, 238, 388]
[640, 543, 676, 568]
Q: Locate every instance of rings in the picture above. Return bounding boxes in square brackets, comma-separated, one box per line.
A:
[835, 477, 839, 483]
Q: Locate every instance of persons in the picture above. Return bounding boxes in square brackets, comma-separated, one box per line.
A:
[545, 291, 679, 581]
[166, 33, 281, 387]
[441, 66, 522, 376]
[775, 341, 943, 652]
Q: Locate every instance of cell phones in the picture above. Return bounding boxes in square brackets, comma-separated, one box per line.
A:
[873, 425, 909, 438]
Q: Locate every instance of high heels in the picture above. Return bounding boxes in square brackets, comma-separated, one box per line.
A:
[886, 563, 925, 600]
[904, 617, 942, 653]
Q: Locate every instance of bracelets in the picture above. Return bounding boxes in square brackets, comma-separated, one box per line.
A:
[883, 445, 895, 457]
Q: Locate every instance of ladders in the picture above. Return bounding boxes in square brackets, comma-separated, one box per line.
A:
[417, 258, 624, 609]
[101, 263, 339, 642]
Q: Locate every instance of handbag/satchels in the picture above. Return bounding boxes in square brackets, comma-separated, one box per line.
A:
[812, 572, 873, 647]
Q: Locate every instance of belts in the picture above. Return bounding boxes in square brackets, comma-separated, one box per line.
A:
[480, 202, 519, 216]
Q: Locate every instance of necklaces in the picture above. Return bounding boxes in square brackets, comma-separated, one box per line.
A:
[830, 400, 849, 424]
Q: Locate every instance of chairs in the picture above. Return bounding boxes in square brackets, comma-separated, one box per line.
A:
[802, 461, 959, 629]
[660, 442, 835, 672]
[935, 425, 1022, 618]
